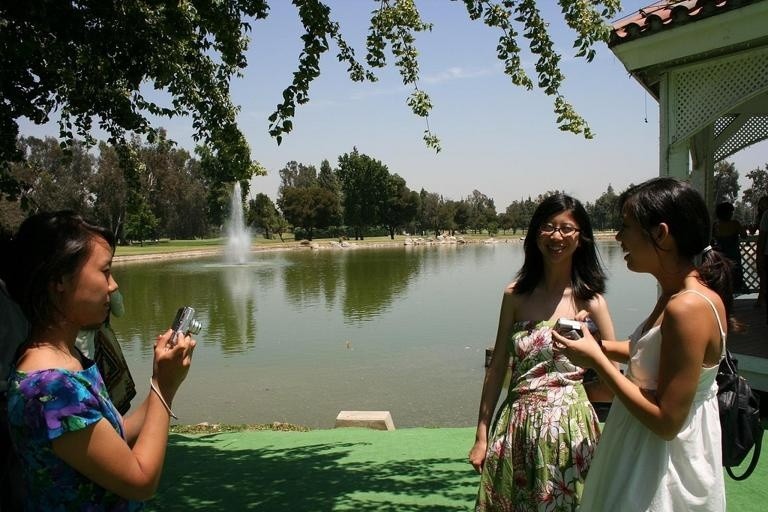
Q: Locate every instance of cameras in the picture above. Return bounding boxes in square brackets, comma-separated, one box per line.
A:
[169, 307, 203, 346]
[554, 318, 598, 338]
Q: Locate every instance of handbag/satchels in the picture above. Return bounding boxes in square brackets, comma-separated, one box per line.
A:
[715, 350, 763, 467]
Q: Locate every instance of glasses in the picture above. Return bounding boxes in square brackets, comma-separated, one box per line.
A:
[540, 224, 579, 239]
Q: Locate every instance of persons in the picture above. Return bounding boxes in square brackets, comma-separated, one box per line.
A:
[550, 175, 743, 512]
[712, 202, 746, 291]
[469, 194, 620, 512]
[0, 209, 196, 512]
[756, 196, 768, 305]
